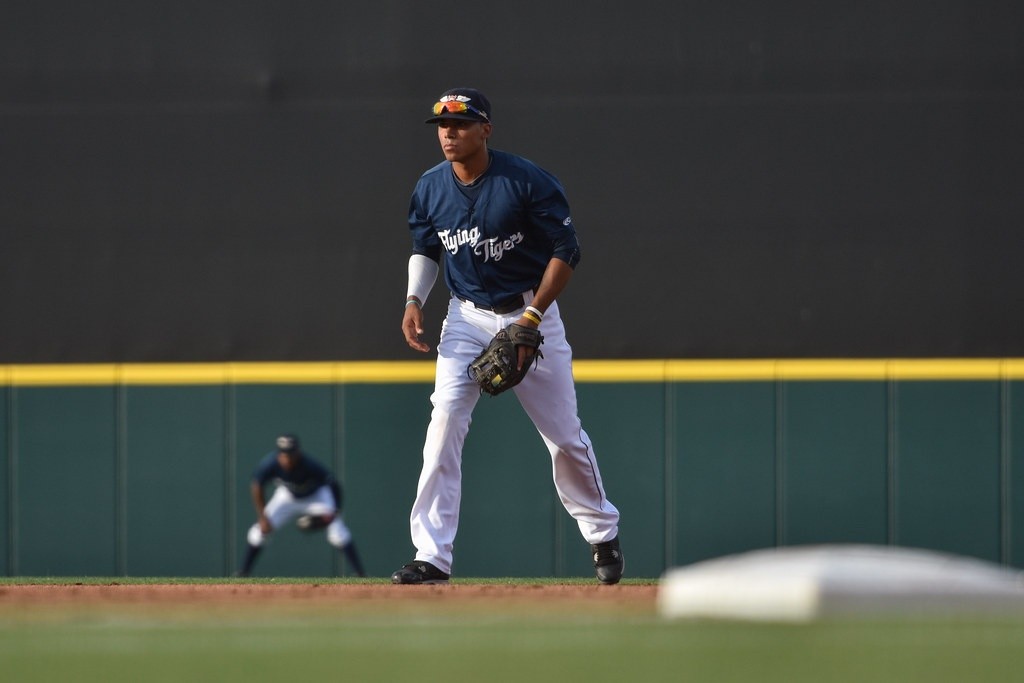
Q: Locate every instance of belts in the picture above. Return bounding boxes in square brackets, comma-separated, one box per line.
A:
[456, 284, 539, 314]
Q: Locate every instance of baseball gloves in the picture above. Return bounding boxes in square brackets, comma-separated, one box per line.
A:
[467, 321, 545, 399]
[295, 514, 328, 532]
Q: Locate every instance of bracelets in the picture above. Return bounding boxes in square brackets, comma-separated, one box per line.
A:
[523, 312, 540, 326]
[405, 300, 421, 310]
[525, 306, 544, 321]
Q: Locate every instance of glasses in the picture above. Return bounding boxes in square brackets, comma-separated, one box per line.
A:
[433, 101, 491, 123]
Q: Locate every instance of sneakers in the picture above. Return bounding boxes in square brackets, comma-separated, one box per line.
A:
[592, 535, 625, 584]
[391, 560, 450, 584]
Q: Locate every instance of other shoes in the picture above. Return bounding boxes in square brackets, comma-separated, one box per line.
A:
[230, 570, 251, 577]
[356, 571, 367, 577]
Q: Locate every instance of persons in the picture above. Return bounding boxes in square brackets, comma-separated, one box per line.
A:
[391, 88, 626, 584]
[230, 433, 367, 578]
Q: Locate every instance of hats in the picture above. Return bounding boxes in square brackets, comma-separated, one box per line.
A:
[276, 434, 300, 453]
[425, 88, 491, 123]
